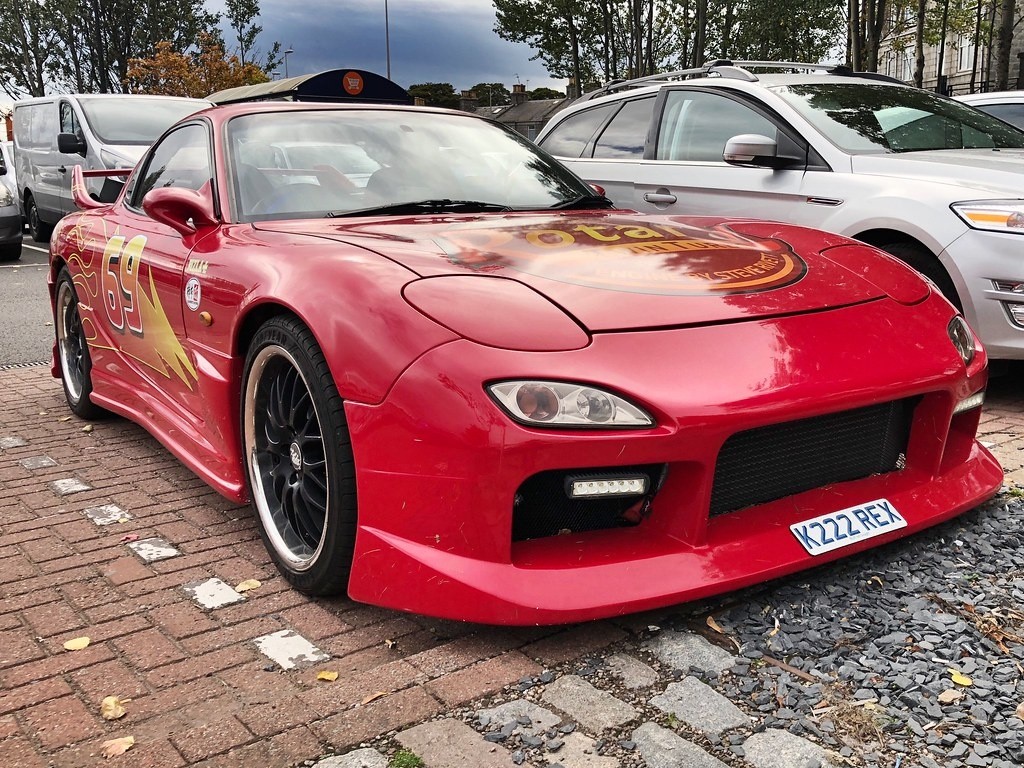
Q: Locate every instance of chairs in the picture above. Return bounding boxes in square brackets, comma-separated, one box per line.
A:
[193, 162, 272, 211]
[367, 166, 427, 198]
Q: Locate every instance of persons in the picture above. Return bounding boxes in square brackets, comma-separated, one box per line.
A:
[360, 130, 438, 203]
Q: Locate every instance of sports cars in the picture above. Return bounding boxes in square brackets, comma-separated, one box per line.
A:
[45, 99, 1008, 627]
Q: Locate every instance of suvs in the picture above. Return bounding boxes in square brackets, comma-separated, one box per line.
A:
[527, 59, 1024, 366]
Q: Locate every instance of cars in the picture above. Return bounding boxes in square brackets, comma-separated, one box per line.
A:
[871, 88, 1024, 152]
[245, 139, 385, 190]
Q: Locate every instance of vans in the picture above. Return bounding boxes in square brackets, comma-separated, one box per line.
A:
[12, 93, 240, 244]
[0, 141, 24, 262]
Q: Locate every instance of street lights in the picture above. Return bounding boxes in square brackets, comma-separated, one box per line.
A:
[284, 49, 294, 78]
[272, 72, 281, 81]
[484, 85, 492, 108]
